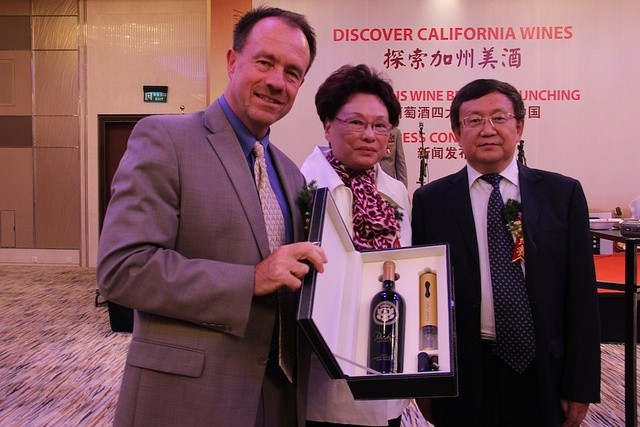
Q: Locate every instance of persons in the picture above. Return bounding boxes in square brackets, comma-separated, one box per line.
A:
[96, 0, 318, 427]
[378, 119, 409, 189]
[297, 61, 412, 426]
[411, 79, 601, 426]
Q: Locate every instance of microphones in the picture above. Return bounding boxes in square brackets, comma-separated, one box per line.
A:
[418, 121, 424, 130]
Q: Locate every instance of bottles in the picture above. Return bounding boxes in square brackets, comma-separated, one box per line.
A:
[367, 260, 403, 374]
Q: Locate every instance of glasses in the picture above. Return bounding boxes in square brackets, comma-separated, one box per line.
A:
[334, 116, 394, 135]
[458, 110, 518, 127]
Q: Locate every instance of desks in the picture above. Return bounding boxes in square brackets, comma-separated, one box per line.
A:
[591, 228, 639, 426]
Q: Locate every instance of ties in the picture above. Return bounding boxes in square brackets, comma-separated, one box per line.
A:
[479, 172, 538, 373]
[251, 141, 296, 384]
